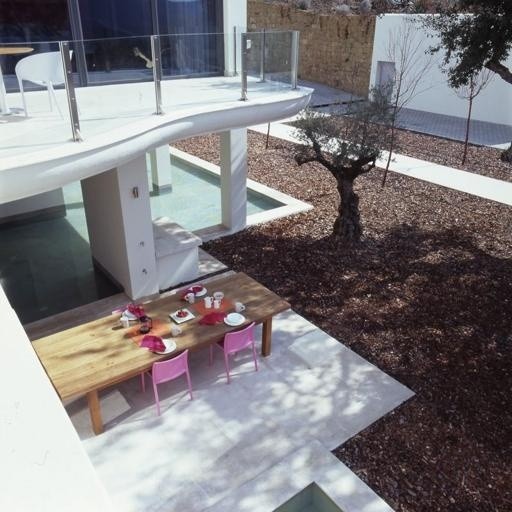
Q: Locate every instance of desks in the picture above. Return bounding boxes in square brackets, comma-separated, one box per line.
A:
[0, 47, 35, 116]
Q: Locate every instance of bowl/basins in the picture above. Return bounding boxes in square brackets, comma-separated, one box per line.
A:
[212, 291, 224, 300]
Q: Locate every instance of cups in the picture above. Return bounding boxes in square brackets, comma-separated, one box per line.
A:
[120, 316, 130, 329]
[235, 302, 245, 313]
[184, 293, 195, 304]
[170, 326, 183, 337]
[213, 299, 222, 310]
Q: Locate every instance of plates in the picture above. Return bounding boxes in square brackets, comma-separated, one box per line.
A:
[153, 339, 177, 354]
[168, 309, 195, 325]
[223, 313, 245, 327]
[188, 285, 208, 297]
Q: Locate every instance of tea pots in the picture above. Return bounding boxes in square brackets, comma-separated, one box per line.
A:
[204, 296, 214, 309]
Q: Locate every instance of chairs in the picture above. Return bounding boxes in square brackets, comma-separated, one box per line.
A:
[15, 50, 74, 116]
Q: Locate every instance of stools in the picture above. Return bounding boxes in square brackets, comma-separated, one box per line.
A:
[147, 213, 202, 291]
